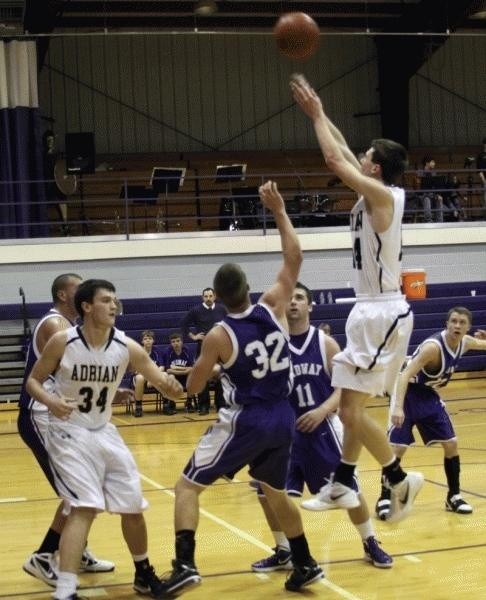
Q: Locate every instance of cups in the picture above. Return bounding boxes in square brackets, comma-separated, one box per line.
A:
[471, 291, 475, 296]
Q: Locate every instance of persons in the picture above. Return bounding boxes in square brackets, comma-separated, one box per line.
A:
[418, 157, 461, 222]
[24, 278, 184, 600]
[180, 287, 228, 418]
[474, 329, 486, 341]
[250, 280, 394, 574]
[160, 179, 327, 597]
[17, 273, 115, 589]
[288, 74, 423, 526]
[474, 137, 486, 187]
[129, 330, 177, 417]
[375, 305, 486, 522]
[317, 322, 331, 336]
[163, 331, 196, 416]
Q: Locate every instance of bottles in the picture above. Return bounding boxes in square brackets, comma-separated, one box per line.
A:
[320, 292, 324, 305]
[327, 292, 333, 304]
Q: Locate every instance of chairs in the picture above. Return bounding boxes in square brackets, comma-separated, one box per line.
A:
[164, 384, 201, 413]
[127, 380, 158, 413]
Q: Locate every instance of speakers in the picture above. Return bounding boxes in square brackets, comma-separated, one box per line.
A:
[64, 131, 97, 176]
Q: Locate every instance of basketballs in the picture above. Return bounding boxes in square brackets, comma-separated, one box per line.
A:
[275, 12, 320, 59]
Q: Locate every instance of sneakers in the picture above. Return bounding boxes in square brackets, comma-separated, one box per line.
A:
[384, 470, 425, 525]
[362, 535, 395, 568]
[251, 545, 295, 573]
[159, 559, 202, 598]
[22, 552, 114, 600]
[299, 471, 361, 512]
[444, 491, 473, 514]
[375, 496, 392, 521]
[135, 399, 210, 417]
[132, 565, 176, 600]
[284, 557, 325, 590]
